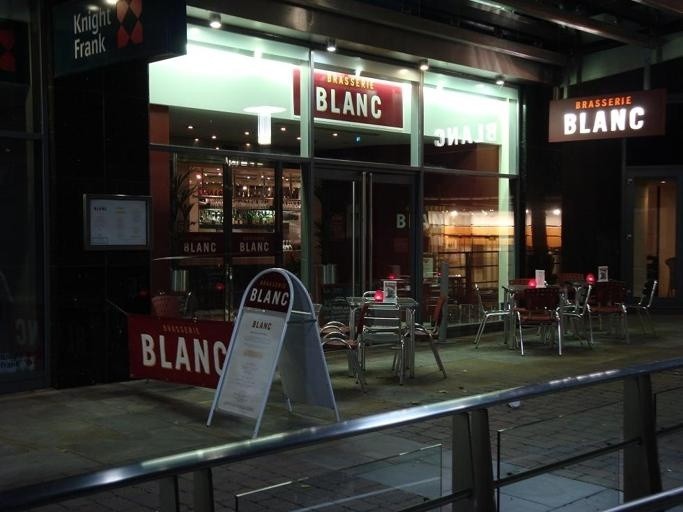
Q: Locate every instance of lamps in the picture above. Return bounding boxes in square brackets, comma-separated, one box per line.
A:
[327, 42, 337, 52]
[497, 75, 504, 86]
[210, 14, 221, 28]
[419, 59, 428, 71]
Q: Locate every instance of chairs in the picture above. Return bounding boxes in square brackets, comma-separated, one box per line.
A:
[474, 278, 658, 357]
[144, 295, 180, 385]
[321, 291, 448, 393]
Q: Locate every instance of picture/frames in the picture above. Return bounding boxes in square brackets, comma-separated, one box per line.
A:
[82, 192, 153, 252]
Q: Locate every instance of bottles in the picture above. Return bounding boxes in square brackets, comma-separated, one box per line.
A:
[198, 209, 275, 227]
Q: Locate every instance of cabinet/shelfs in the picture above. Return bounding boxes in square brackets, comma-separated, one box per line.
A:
[198, 194, 300, 229]
